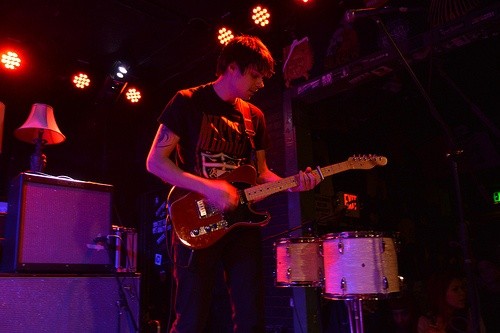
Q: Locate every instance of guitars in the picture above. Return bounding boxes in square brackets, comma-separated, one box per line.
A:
[166, 152, 388, 250]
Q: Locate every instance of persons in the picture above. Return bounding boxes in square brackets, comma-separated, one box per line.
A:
[417, 275, 485, 333]
[145, 33, 321, 333]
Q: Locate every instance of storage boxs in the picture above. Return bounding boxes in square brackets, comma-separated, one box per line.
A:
[114, 227, 138, 273]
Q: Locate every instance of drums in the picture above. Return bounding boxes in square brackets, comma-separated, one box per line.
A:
[321, 230, 403, 301]
[273, 236, 324, 288]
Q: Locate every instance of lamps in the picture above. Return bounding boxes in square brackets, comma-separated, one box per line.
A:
[14, 102, 67, 173]
